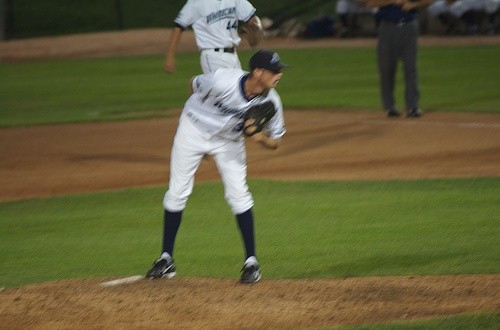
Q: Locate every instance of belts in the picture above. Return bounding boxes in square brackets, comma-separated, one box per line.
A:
[390, 19, 414, 24]
[215, 48, 235, 53]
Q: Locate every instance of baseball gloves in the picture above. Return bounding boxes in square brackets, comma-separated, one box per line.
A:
[242, 101, 276, 138]
[245, 16, 263, 50]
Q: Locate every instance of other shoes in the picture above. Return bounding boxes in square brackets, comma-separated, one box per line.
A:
[388, 109, 402, 117]
[408, 108, 423, 117]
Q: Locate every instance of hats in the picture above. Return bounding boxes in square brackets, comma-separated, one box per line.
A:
[249, 50, 292, 73]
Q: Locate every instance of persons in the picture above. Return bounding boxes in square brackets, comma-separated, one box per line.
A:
[336, 0, 500, 36]
[145, 49, 292, 285]
[366, 0, 432, 117]
[163, 0, 263, 75]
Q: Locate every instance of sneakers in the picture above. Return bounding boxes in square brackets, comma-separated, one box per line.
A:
[146, 257, 177, 281]
[240, 263, 262, 283]
